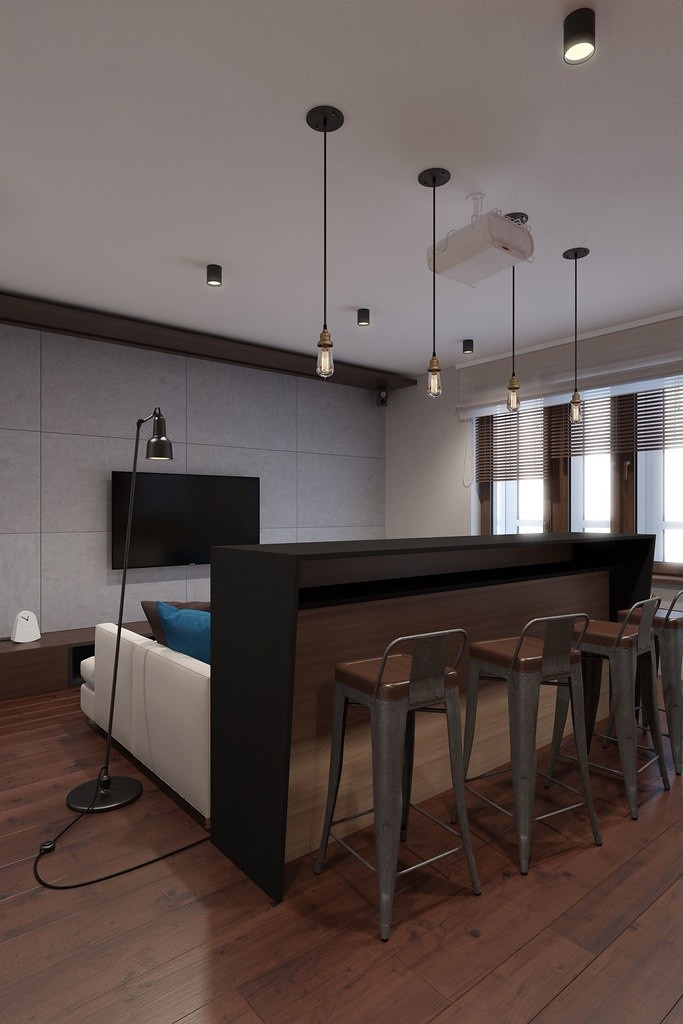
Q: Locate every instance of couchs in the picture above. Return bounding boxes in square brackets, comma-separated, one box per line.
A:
[75, 621, 211, 830]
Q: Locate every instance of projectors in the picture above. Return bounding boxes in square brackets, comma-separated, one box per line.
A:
[426, 213, 535, 285]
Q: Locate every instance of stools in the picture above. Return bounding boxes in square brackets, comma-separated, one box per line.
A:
[541, 595, 671, 821]
[460, 613, 603, 874]
[314, 627, 484, 942]
[616, 581, 683, 778]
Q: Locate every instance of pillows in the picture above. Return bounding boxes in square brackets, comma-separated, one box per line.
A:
[155, 601, 211, 662]
[141, 599, 210, 645]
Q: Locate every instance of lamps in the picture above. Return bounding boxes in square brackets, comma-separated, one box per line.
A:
[304, 107, 344, 377]
[498, 212, 526, 411]
[420, 169, 452, 396]
[358, 307, 370, 327]
[66, 405, 173, 815]
[461, 339, 474, 355]
[563, 8, 597, 64]
[561, 249, 588, 422]
[206, 266, 221, 287]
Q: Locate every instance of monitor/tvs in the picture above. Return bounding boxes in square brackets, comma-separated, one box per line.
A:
[112, 470, 260, 570]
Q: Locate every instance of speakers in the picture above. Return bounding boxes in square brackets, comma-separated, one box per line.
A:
[377, 390, 388, 406]
